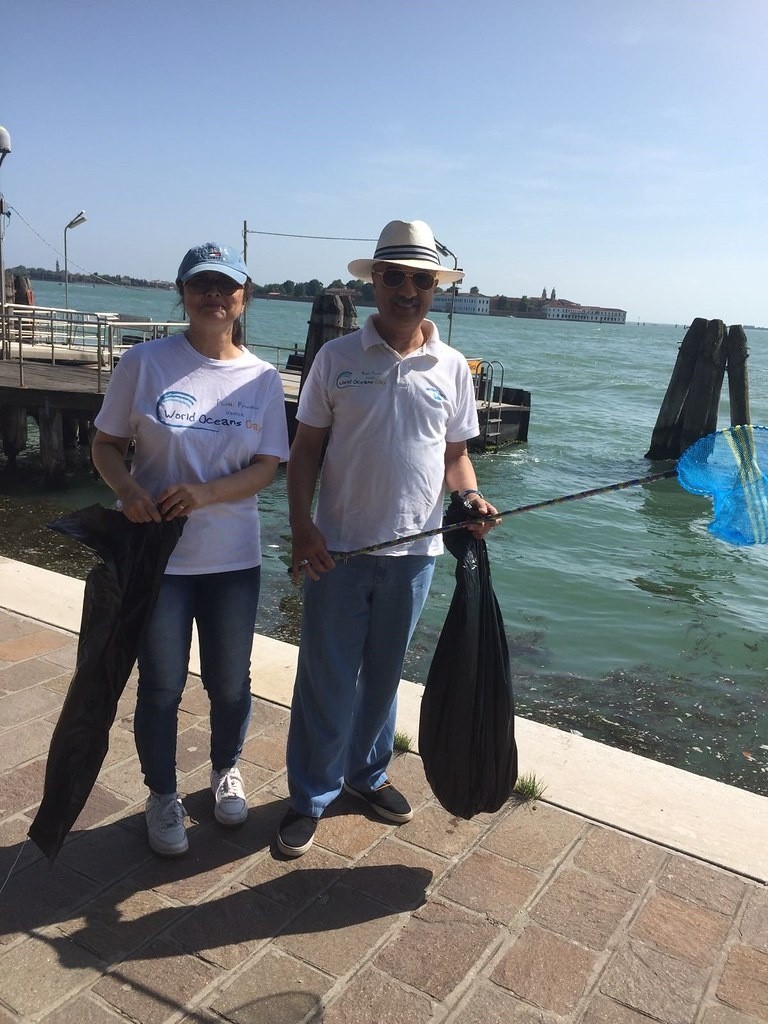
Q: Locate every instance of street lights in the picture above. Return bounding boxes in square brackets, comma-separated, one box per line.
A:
[434, 237, 457, 347]
[64, 211, 87, 341]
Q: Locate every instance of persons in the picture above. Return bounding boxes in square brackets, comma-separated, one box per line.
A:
[275, 222, 502, 855]
[91, 240, 290, 856]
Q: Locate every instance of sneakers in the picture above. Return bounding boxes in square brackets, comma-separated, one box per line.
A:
[145, 788, 190, 858]
[277, 806, 319, 857]
[344, 778, 414, 823]
[211, 766, 248, 828]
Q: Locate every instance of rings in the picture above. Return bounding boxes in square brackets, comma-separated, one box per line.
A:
[177, 503, 184, 511]
[298, 560, 309, 566]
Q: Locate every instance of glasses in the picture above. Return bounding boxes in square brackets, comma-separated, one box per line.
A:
[186, 277, 245, 296]
[372, 268, 437, 292]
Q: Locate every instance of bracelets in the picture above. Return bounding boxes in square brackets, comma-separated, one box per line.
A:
[460, 489, 484, 498]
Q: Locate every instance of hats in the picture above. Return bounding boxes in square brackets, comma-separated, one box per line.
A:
[347, 219, 465, 285]
[177, 242, 252, 287]
